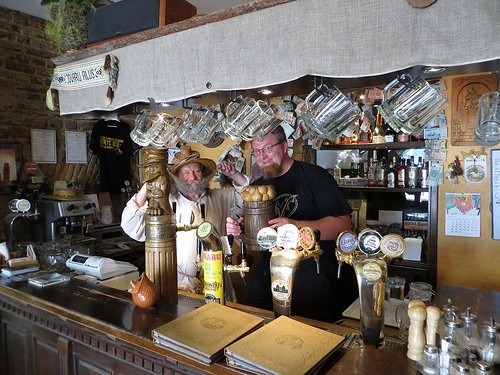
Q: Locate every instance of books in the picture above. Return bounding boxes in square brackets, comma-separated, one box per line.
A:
[224, 315, 346, 375]
[151, 302, 265, 366]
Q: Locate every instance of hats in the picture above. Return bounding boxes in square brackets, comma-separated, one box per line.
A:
[169, 151, 216, 184]
[41, 180, 89, 201]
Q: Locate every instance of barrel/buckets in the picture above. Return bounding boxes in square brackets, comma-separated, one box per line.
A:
[241, 196, 275, 250]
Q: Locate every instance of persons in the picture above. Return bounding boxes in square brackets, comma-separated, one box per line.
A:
[225, 124, 360, 324]
[121, 150, 250, 292]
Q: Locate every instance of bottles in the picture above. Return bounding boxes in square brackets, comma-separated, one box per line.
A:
[406, 297, 500, 375]
[324, 110, 429, 189]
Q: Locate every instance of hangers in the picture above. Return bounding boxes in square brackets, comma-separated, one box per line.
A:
[93, 112, 133, 133]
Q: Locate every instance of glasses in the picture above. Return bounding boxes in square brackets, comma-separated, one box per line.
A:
[251, 141, 284, 157]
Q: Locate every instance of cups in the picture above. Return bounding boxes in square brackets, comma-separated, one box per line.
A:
[128, 71, 448, 149]
[383, 277, 432, 341]
[475, 92, 500, 144]
[14, 238, 91, 273]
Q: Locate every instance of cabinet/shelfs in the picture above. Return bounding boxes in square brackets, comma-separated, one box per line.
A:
[300, 141, 431, 270]
